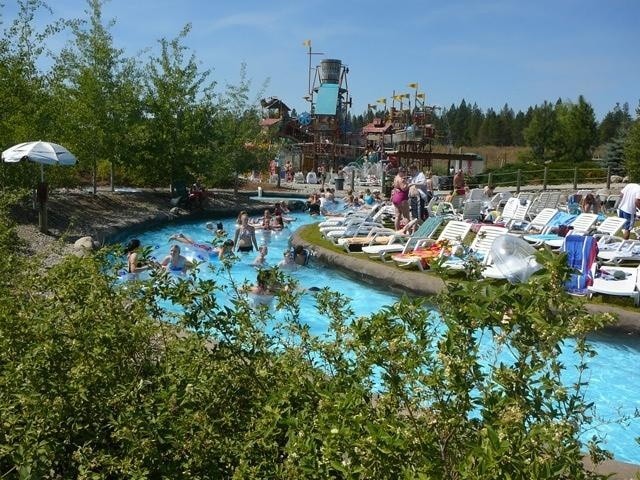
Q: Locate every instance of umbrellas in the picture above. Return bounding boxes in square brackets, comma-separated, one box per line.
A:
[1, 139, 80, 234]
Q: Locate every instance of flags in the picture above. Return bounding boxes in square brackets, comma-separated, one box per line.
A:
[369, 82, 425, 112]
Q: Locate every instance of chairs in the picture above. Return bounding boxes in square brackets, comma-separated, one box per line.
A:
[390, 221, 508, 274]
[587, 239, 640, 305]
[427, 187, 504, 219]
[318, 203, 444, 258]
[493, 189, 627, 250]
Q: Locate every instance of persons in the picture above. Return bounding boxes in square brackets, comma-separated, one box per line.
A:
[389, 164, 463, 230]
[581, 193, 599, 214]
[284, 161, 292, 183]
[126, 183, 381, 297]
[270, 158, 276, 177]
[616, 182, 640, 241]
[315, 163, 327, 185]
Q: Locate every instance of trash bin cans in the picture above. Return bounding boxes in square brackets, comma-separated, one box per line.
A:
[334, 178, 344, 190]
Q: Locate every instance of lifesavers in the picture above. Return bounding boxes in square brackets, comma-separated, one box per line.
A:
[491, 234, 544, 286]
[193, 242, 232, 262]
